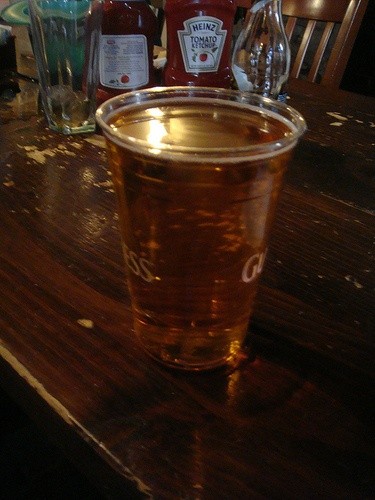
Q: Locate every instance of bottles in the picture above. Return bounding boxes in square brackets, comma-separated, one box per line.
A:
[82, 0, 157, 110]
[231, 0, 291, 98]
[164, 0, 237, 99]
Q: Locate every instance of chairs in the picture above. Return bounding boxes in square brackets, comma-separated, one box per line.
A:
[244, 0, 368, 89]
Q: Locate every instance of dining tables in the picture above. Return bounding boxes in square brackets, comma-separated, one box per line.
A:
[0, 76, 375, 499]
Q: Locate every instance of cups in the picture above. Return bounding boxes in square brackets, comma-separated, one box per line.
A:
[95, 84, 307, 371]
[26, 0, 102, 134]
